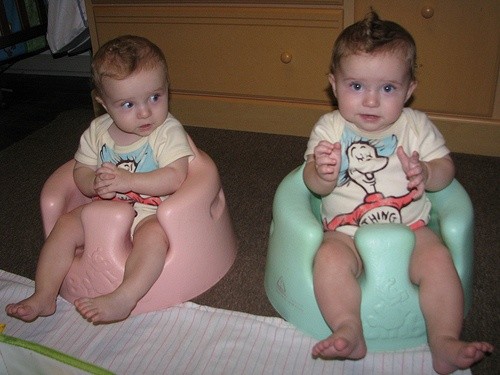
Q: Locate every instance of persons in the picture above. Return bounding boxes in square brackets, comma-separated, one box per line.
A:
[5, 34, 197, 326]
[301, 5, 496, 375]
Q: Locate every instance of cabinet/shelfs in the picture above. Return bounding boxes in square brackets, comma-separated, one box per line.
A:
[354, 0, 500, 156]
[86, 0, 352, 137]
